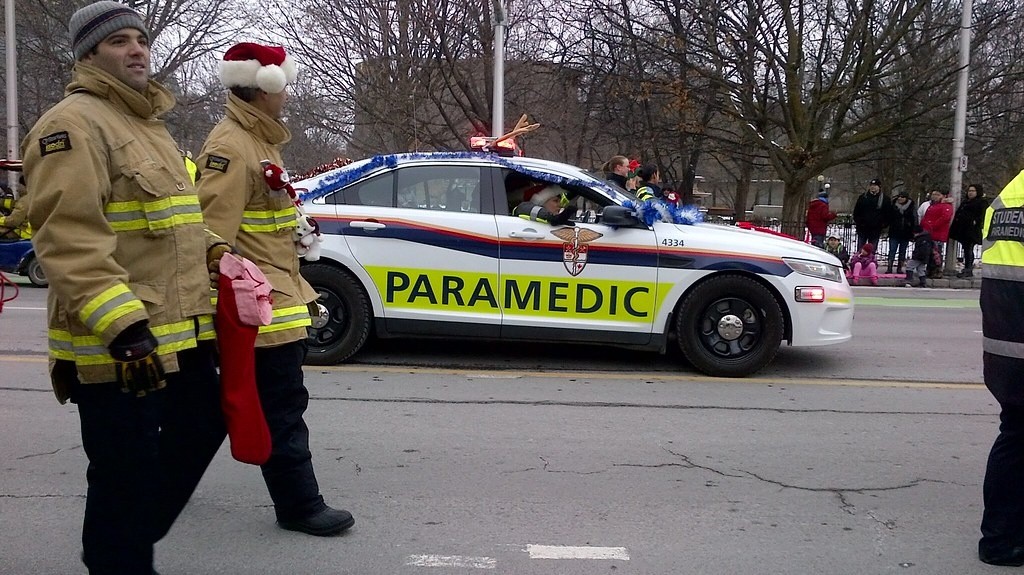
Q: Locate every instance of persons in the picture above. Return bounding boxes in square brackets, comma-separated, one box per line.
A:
[601, 155, 683, 210]
[189, 41, 355, 537]
[1, 174, 32, 242]
[504, 176, 578, 225]
[376, 185, 416, 209]
[18, 0, 240, 575]
[806, 180, 987, 287]
[978, 168, 1023, 566]
[446, 183, 466, 211]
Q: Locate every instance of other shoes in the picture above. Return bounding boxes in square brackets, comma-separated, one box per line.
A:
[871, 278, 877, 285]
[280, 504, 355, 537]
[852, 276, 859, 285]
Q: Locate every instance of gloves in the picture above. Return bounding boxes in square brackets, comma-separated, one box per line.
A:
[206, 244, 243, 290]
[108, 320, 168, 402]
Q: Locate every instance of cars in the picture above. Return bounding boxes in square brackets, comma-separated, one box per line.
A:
[289, 137, 854, 378]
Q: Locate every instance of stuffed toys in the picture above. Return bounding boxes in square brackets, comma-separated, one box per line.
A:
[293, 215, 324, 261]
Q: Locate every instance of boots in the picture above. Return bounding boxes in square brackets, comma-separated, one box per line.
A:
[904, 270, 914, 280]
[884, 259, 893, 274]
[918, 276, 927, 287]
[897, 259, 904, 274]
[956, 260, 974, 277]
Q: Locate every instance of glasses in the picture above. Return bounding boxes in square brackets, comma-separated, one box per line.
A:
[967, 190, 976, 192]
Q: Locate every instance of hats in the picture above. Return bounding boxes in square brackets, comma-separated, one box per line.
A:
[870, 178, 882, 188]
[828, 233, 840, 239]
[899, 191, 909, 196]
[219, 42, 299, 94]
[17, 175, 26, 192]
[816, 188, 828, 198]
[523, 184, 562, 205]
[69, 1, 150, 61]
[862, 243, 874, 253]
